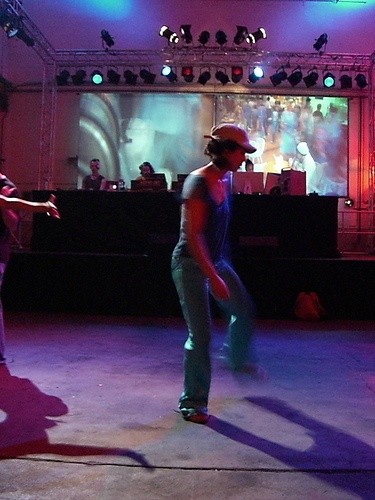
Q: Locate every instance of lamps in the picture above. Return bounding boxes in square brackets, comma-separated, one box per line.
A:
[230, 66, 243, 84]
[123, 70, 138, 86]
[179, 24, 193, 44]
[247, 66, 265, 85]
[214, 66, 231, 86]
[55, 69, 70, 86]
[181, 66, 195, 83]
[269, 65, 287, 87]
[354, 72, 368, 90]
[139, 66, 156, 84]
[101, 30, 115, 48]
[322, 65, 336, 88]
[89, 70, 105, 87]
[215, 30, 228, 47]
[197, 30, 210, 46]
[107, 69, 121, 84]
[287, 65, 303, 88]
[233, 25, 248, 46]
[196, 66, 211, 86]
[71, 70, 87, 86]
[0, 18, 18, 38]
[246, 26, 267, 44]
[339, 75, 353, 89]
[160, 64, 178, 84]
[312, 32, 328, 53]
[303, 64, 319, 88]
[158, 25, 179, 46]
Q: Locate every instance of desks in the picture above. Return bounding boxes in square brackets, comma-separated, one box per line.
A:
[23, 190, 344, 257]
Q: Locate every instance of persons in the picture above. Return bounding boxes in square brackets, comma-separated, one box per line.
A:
[83, 159, 105, 189]
[172, 124, 257, 424]
[137, 162, 154, 180]
[113, 85, 347, 197]
[246, 159, 255, 172]
[0, 158, 60, 364]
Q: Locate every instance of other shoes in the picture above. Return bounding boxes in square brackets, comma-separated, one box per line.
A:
[218, 358, 264, 381]
[184, 410, 208, 423]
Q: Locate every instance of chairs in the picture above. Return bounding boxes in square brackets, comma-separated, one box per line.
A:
[177, 174, 190, 181]
[154, 173, 168, 190]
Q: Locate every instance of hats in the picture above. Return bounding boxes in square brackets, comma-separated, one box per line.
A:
[203, 123, 257, 154]
